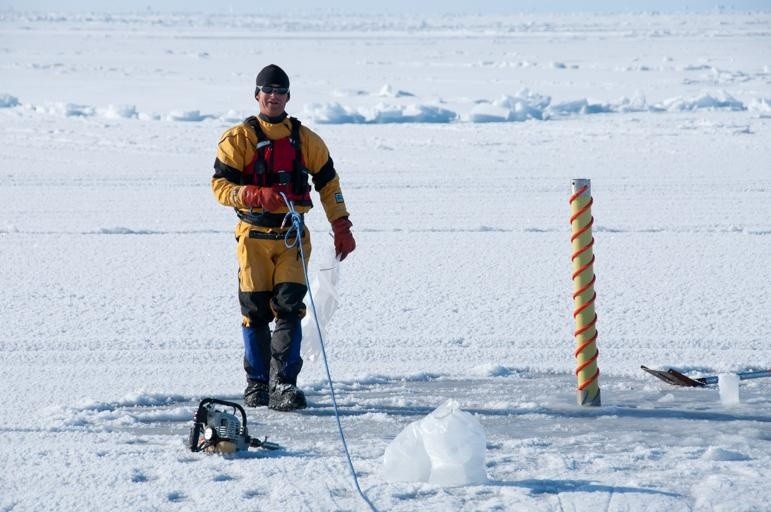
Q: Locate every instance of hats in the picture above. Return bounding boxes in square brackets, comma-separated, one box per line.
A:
[255, 64, 291, 88]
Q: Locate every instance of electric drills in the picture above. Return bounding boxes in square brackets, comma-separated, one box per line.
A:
[194, 398, 288, 455]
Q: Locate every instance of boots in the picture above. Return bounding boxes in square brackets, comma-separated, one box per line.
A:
[245, 382, 269, 407]
[271, 382, 308, 411]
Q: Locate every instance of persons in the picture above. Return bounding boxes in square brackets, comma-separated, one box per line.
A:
[210, 63, 355, 413]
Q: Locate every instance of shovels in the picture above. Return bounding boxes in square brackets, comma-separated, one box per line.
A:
[640, 366, 770, 387]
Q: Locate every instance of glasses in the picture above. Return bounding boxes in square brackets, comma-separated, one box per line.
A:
[260, 83, 288, 95]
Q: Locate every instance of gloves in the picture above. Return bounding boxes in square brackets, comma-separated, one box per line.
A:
[242, 185, 284, 210]
[332, 217, 357, 262]
